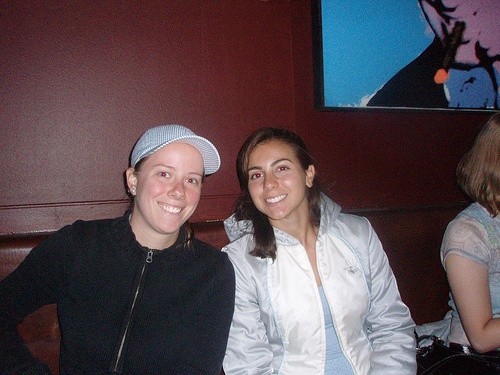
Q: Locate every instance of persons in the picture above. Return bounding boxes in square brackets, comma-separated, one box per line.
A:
[440, 114, 500, 353]
[220, 126, 417, 375]
[0, 124, 236, 375]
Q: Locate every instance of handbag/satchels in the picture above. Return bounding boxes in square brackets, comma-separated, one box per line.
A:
[416, 334, 500, 375]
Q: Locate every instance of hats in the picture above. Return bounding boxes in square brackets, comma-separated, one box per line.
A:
[130, 124, 221, 179]
